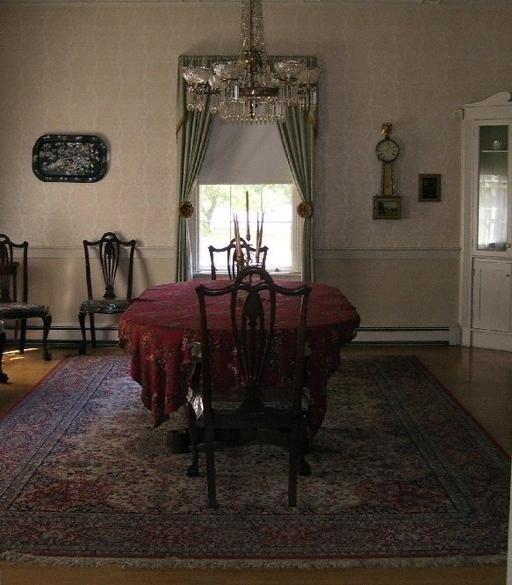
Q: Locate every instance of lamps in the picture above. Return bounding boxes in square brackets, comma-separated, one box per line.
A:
[177, 0, 322, 126]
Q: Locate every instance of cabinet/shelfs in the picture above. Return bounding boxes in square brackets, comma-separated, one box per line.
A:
[454, 89, 512, 352]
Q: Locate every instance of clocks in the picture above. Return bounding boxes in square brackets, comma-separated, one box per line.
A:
[376, 122, 399, 194]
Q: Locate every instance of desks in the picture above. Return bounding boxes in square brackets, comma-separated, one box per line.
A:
[117, 281, 361, 451]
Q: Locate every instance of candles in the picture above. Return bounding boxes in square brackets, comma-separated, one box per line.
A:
[232, 210, 265, 257]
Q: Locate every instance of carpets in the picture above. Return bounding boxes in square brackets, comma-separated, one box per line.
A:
[0, 350, 510, 570]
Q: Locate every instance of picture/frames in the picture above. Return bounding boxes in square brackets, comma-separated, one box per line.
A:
[30, 132, 108, 183]
[418, 173, 442, 202]
[372, 195, 402, 220]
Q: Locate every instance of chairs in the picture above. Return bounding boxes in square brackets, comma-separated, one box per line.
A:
[0, 233, 52, 365]
[208, 238, 268, 281]
[185, 269, 315, 508]
[77, 231, 136, 354]
